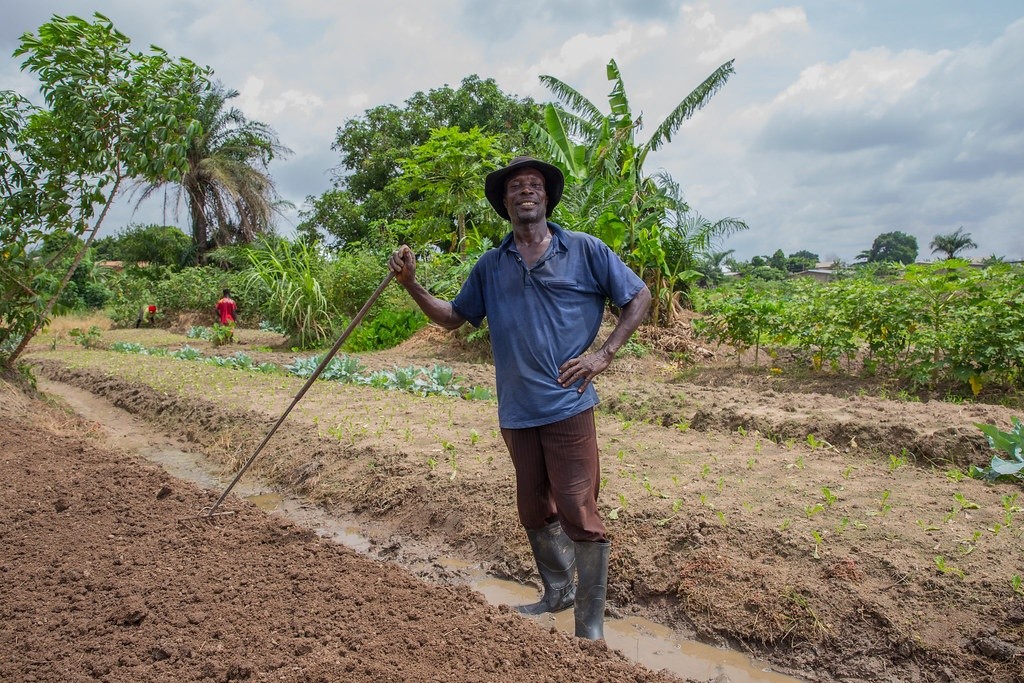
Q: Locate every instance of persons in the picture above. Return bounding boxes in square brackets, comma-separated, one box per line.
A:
[136, 304, 156, 328]
[215, 289, 237, 325]
[388, 156, 652, 641]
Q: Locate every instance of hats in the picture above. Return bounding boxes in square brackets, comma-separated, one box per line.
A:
[485, 156, 564, 222]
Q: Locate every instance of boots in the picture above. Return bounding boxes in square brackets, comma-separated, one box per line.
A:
[574, 539, 610, 644]
[514, 520, 576, 616]
